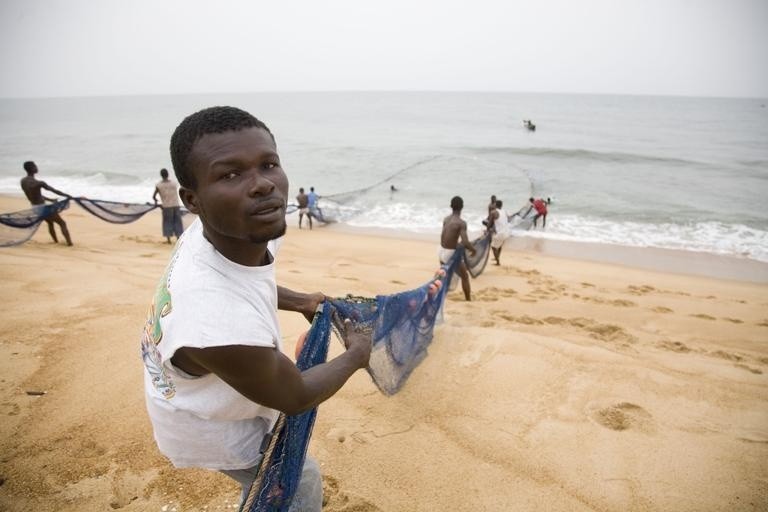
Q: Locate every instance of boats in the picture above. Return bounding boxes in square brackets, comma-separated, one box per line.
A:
[523, 119, 535, 130]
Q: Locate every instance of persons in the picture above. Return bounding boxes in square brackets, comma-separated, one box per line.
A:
[20, 162, 73, 246]
[296, 188, 312, 229]
[140, 106, 375, 512]
[307, 188, 323, 221]
[439, 196, 477, 301]
[391, 186, 398, 191]
[522, 198, 548, 229]
[153, 168, 183, 244]
[487, 200, 509, 265]
[488, 196, 496, 214]
